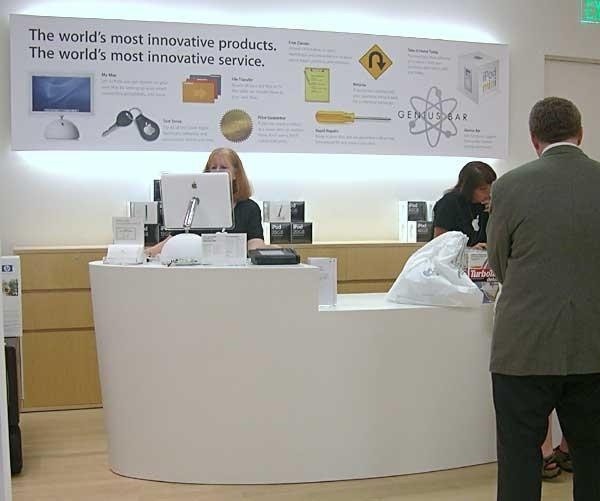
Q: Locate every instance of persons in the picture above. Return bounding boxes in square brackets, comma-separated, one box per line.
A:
[143, 147, 284, 258]
[432, 160, 498, 249]
[487, 97, 600, 499]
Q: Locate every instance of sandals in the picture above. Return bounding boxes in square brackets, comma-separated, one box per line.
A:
[542, 455, 562, 479]
[554, 445, 574, 474]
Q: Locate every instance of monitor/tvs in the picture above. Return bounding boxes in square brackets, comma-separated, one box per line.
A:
[31, 75, 93, 140]
[161, 172, 234, 264]
